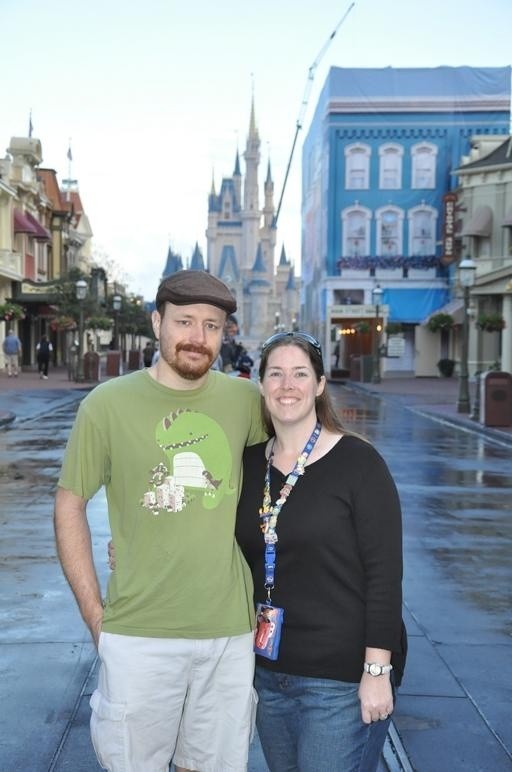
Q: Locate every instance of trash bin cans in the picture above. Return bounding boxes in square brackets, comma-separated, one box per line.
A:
[106, 351, 121, 377]
[468, 370, 512, 426]
[83, 351, 99, 384]
[349, 354, 374, 383]
[128, 350, 140, 370]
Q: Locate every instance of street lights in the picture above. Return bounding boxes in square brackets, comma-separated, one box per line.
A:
[372, 284, 385, 385]
[458, 253, 477, 414]
[113, 295, 122, 350]
[75, 277, 88, 382]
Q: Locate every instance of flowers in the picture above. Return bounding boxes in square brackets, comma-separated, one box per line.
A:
[0, 303, 27, 321]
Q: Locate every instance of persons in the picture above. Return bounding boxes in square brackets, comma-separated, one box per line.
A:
[141, 339, 256, 379]
[332, 340, 340, 367]
[36, 335, 54, 380]
[106, 329, 408, 771]
[52, 268, 274, 772]
[3, 329, 23, 377]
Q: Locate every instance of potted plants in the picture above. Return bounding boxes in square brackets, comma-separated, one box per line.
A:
[435, 359, 456, 377]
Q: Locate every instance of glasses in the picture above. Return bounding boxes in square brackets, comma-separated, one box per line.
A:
[259, 331, 323, 351]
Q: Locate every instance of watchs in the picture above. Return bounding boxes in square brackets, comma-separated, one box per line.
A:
[362, 661, 393, 676]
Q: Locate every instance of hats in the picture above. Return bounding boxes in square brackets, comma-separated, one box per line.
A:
[156, 269, 238, 321]
